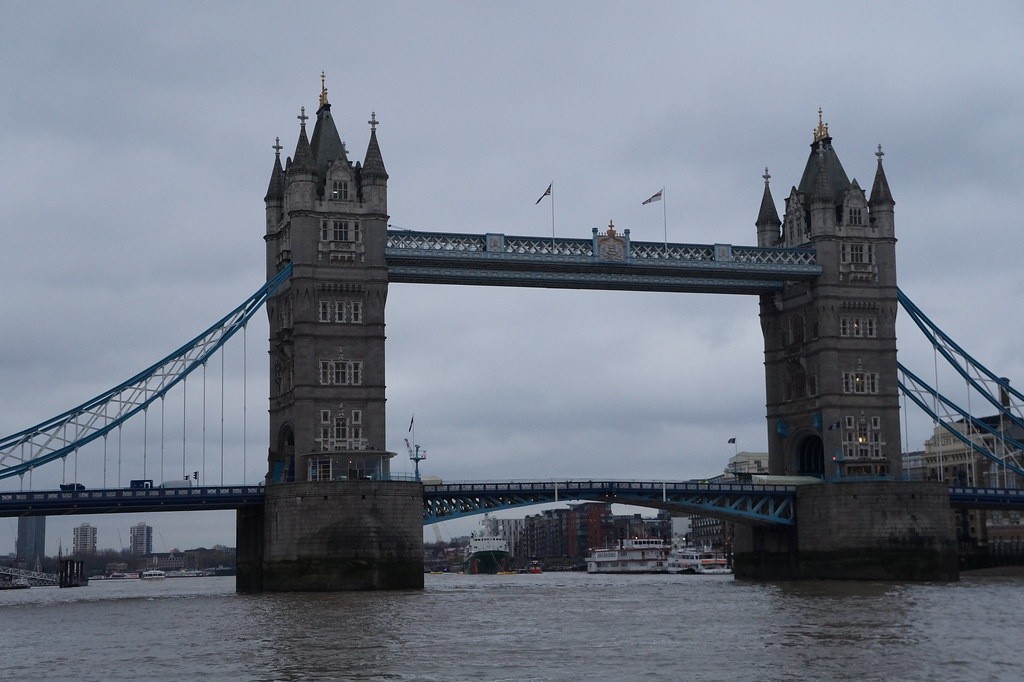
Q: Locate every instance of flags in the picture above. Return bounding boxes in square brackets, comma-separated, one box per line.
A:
[535, 184, 551, 204]
[728, 438, 735, 443]
[642, 189, 663, 205]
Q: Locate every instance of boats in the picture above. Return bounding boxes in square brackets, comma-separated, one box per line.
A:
[430, 511, 544, 574]
[584, 523, 727, 573]
